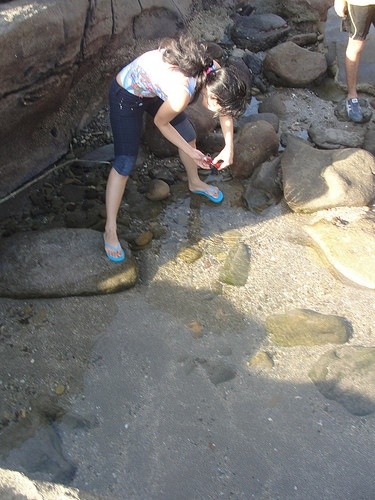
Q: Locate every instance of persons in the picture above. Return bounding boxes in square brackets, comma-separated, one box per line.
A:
[334, 0, 375, 121]
[103, 38, 246, 261]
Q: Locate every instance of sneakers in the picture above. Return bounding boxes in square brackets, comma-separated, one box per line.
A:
[345, 97, 364, 122]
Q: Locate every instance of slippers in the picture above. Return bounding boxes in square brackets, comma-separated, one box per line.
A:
[192, 185, 224, 203]
[104, 242, 125, 262]
